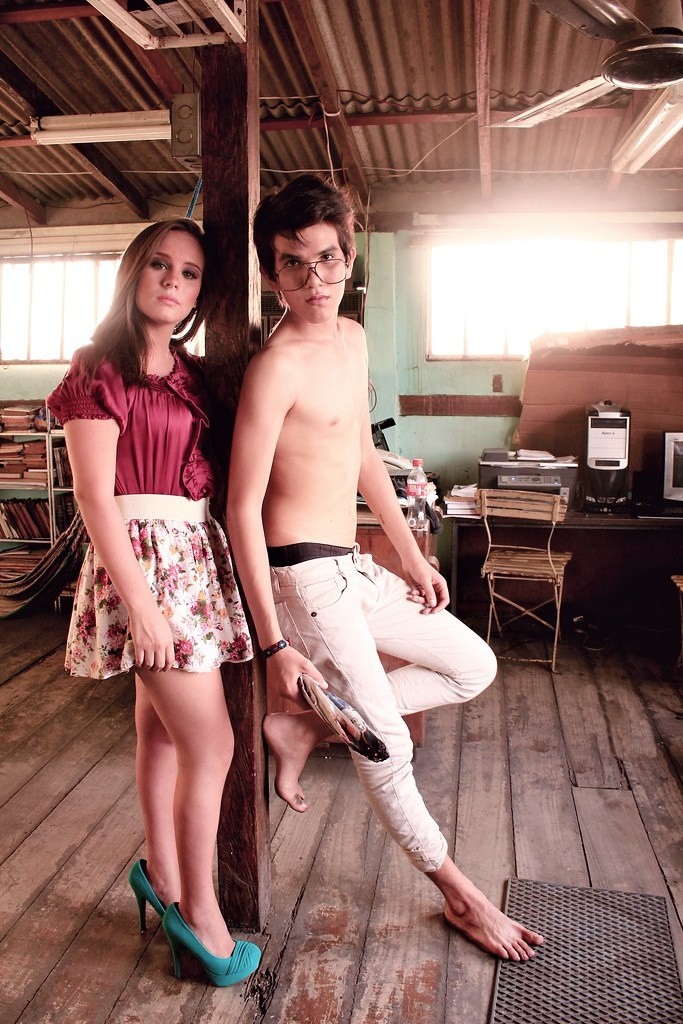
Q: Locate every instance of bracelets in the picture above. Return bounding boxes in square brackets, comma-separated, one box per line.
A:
[263, 638, 293, 658]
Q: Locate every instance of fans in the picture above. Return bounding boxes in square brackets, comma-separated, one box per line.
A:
[485, 0, 683, 174]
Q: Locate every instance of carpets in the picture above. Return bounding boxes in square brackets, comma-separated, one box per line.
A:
[488, 876, 683, 1024]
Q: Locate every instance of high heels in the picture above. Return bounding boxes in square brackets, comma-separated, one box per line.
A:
[129, 858, 168, 932]
[163, 903, 261, 987]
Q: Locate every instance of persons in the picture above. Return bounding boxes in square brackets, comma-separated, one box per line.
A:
[46, 218, 262, 986]
[225, 172, 543, 962]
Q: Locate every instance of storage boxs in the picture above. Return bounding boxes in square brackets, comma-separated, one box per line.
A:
[478, 465, 579, 511]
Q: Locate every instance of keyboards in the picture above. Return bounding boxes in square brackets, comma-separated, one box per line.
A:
[662, 507, 683, 517]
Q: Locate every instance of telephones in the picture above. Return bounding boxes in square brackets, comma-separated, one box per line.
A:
[375, 447, 414, 471]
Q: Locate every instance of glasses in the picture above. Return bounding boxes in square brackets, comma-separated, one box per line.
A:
[269, 253, 351, 292]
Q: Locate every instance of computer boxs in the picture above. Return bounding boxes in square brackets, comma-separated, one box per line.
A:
[582, 399, 631, 514]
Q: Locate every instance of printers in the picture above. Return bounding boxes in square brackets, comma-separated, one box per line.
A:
[478, 448, 581, 513]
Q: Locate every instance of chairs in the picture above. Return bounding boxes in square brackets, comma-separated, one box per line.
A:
[475, 487, 573, 673]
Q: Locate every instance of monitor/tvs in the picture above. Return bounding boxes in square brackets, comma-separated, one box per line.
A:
[663, 433, 683, 501]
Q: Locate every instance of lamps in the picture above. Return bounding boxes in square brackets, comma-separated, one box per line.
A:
[31, 109, 171, 145]
[613, 84, 683, 173]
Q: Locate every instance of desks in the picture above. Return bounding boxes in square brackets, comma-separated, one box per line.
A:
[451, 511, 683, 617]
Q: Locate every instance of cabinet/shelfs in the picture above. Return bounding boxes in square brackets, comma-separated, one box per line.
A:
[0, 400, 91, 612]
[265, 519, 434, 750]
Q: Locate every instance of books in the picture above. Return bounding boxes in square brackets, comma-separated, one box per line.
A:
[0, 404, 91, 596]
[444, 484, 477, 515]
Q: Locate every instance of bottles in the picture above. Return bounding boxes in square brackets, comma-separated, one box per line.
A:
[407, 459, 428, 529]
